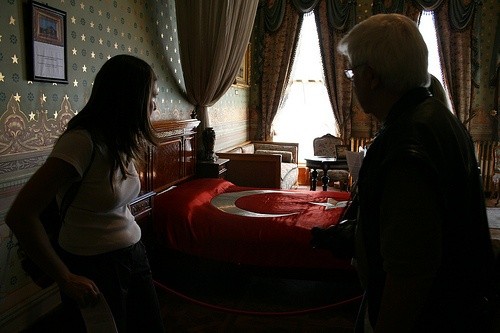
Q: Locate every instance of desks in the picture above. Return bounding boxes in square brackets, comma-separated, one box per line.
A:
[306, 155, 348, 190]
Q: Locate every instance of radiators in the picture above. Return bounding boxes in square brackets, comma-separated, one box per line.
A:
[350, 136, 500, 193]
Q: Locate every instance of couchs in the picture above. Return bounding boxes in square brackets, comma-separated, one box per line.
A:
[216, 141, 300, 192]
[306, 134, 349, 188]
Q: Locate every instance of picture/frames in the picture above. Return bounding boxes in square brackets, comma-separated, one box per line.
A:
[232, 42, 251, 88]
[22, 0, 70, 84]
[334, 145, 350, 160]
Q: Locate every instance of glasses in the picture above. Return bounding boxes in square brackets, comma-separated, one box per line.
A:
[343, 64, 374, 82]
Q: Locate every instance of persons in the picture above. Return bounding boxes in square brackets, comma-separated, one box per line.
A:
[4, 55, 164, 333]
[309, 13, 500, 333]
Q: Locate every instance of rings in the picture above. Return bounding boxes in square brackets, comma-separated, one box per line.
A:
[84, 293, 89, 297]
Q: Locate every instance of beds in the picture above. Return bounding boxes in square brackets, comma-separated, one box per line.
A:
[152, 175, 353, 272]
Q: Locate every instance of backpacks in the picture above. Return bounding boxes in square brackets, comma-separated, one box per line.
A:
[21, 198, 60, 287]
